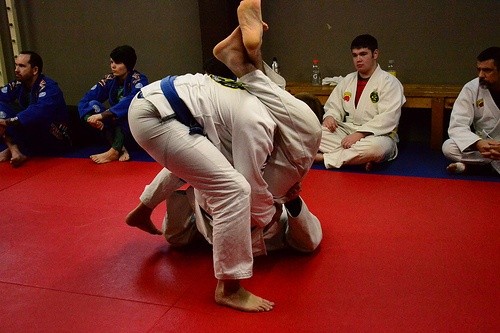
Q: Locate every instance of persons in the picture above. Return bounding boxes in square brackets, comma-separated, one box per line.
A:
[312, 34, 406, 171]
[77, 45, 149, 164]
[442, 47, 500, 174]
[125, 1, 323, 312]
[0, 51, 68, 167]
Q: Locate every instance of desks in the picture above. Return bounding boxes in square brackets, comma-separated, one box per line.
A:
[286, 81, 465, 148]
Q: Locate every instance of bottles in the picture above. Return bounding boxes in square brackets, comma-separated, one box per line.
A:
[387, 59, 396, 78]
[310, 59, 322, 86]
[270, 57, 281, 75]
